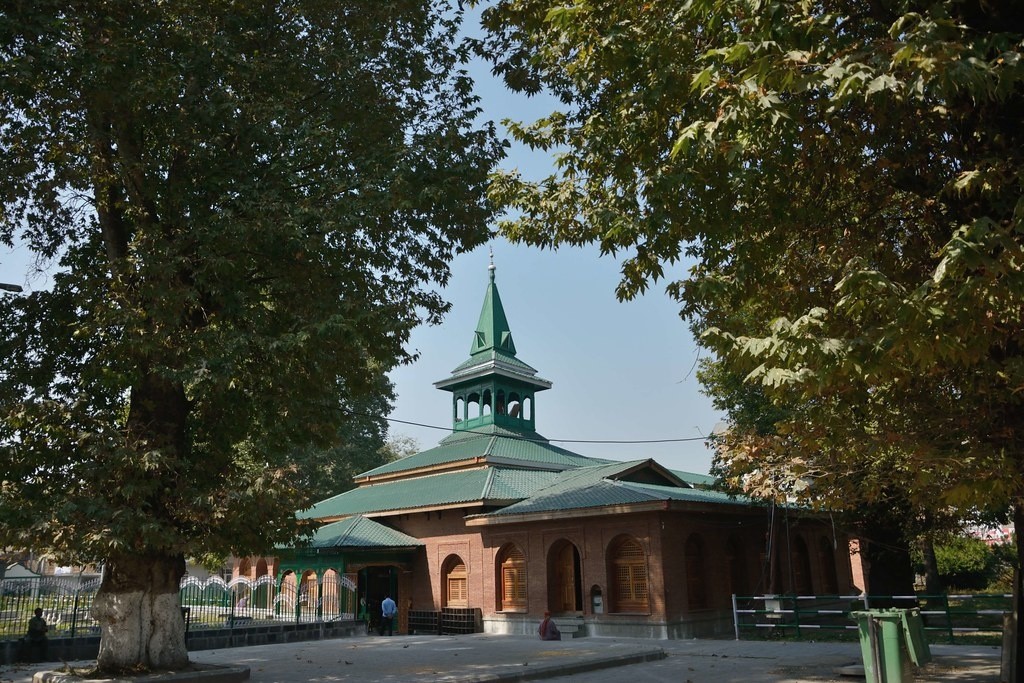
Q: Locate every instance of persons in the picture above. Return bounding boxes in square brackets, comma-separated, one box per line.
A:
[380, 590, 398, 635]
[536, 611, 560, 640]
[26, 606, 51, 660]
[238, 597, 249, 608]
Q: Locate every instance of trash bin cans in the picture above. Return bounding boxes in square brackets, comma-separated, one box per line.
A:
[849, 607, 931, 683]
